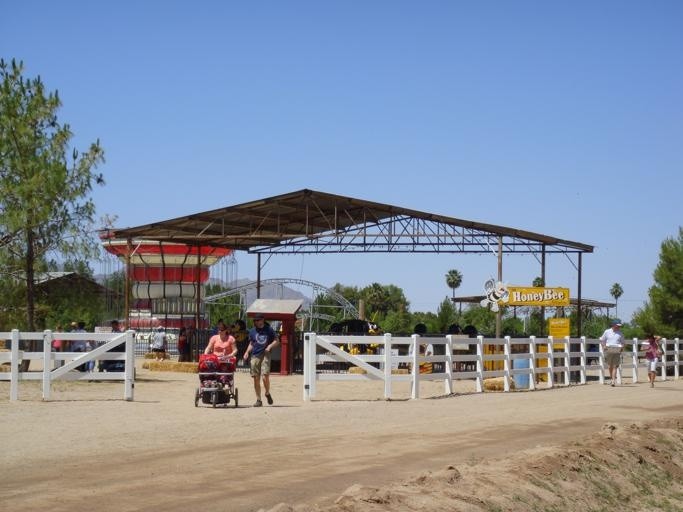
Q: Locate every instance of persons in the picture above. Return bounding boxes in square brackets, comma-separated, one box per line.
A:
[152, 326, 168, 361]
[348, 344, 374, 356]
[203, 324, 239, 408]
[598, 320, 626, 386]
[640, 333, 663, 388]
[52, 317, 123, 383]
[177, 328, 190, 362]
[216, 318, 249, 335]
[243, 313, 279, 406]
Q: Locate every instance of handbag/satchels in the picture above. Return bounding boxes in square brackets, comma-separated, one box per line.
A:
[656, 344, 664, 355]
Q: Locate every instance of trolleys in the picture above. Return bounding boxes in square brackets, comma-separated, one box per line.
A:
[194, 369, 239, 409]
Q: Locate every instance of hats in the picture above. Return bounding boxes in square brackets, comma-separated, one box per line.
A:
[253, 314, 264, 321]
[612, 320, 622, 326]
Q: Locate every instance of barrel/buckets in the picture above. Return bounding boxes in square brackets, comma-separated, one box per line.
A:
[512, 359, 530, 389]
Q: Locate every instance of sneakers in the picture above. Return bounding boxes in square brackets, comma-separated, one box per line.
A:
[253, 400, 262, 407]
[265, 392, 273, 405]
